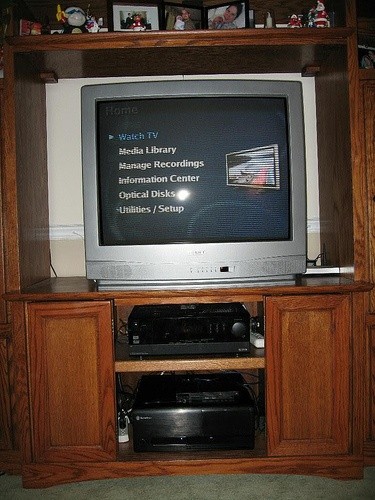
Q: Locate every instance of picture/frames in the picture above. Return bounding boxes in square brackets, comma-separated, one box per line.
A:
[109, 1, 164, 31]
[159, 1, 201, 31]
[201, 1, 248, 29]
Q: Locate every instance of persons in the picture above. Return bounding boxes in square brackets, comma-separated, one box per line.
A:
[210, 3, 243, 30]
[288, 13, 303, 29]
[126, 12, 144, 29]
[311, 1, 331, 28]
[174, 8, 196, 30]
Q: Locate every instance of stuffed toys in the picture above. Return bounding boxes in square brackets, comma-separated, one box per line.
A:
[55, 4, 91, 35]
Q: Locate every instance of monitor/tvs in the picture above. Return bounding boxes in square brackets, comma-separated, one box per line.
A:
[80, 79, 309, 289]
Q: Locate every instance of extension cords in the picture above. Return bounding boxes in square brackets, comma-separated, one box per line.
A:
[117, 413, 131, 445]
[249, 328, 266, 350]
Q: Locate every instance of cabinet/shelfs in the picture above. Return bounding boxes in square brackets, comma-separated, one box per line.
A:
[2, 0, 371, 489]
